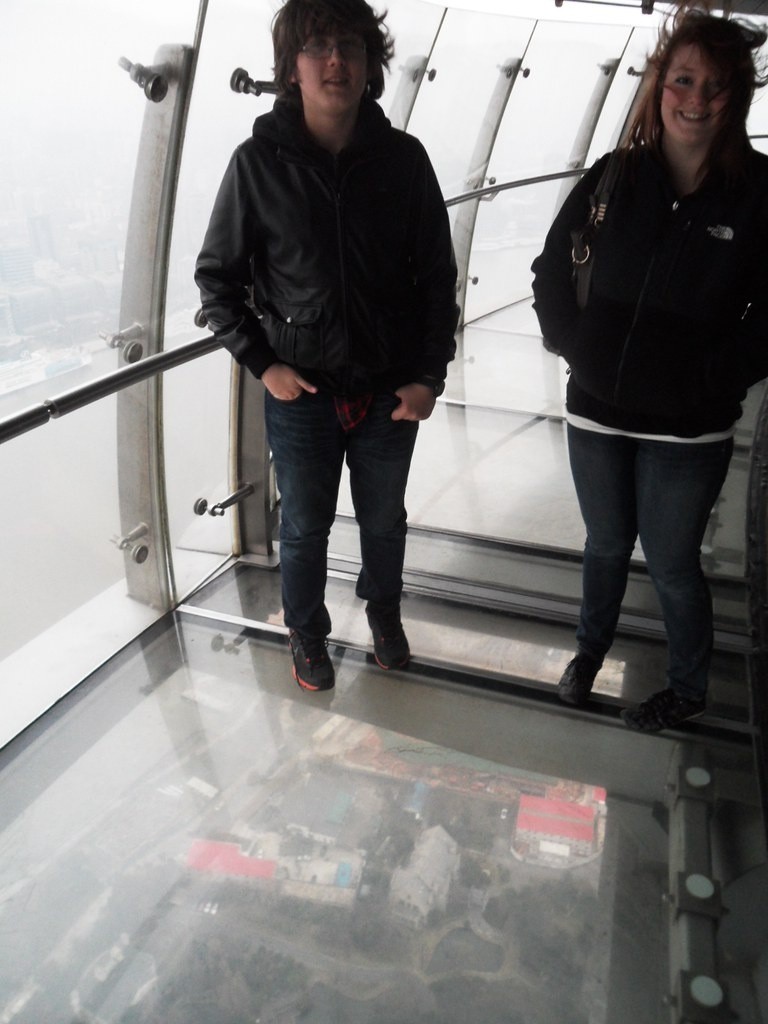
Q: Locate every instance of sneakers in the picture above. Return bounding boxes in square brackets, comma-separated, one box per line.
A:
[621, 689, 706, 733]
[366, 604, 410, 670]
[557, 642, 604, 703]
[289, 628, 335, 691]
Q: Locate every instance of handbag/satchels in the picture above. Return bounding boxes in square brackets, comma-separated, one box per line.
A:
[569, 222, 623, 312]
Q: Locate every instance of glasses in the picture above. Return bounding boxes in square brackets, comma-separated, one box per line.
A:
[299, 34, 365, 59]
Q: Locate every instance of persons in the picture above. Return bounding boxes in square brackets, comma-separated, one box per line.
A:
[194, 0, 460, 691]
[529, 13, 768, 732]
